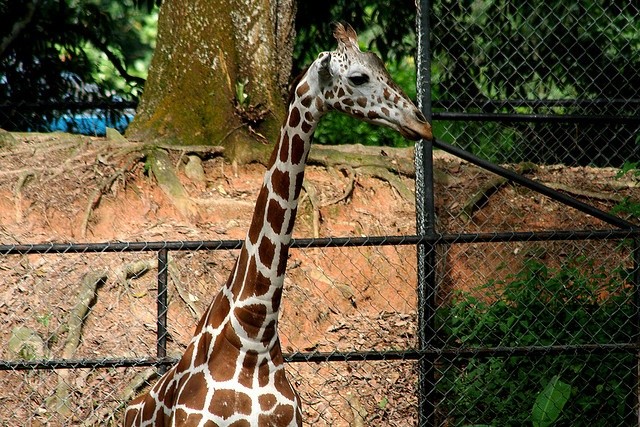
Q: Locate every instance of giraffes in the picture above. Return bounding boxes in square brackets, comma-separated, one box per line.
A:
[118, 19, 435, 427]
